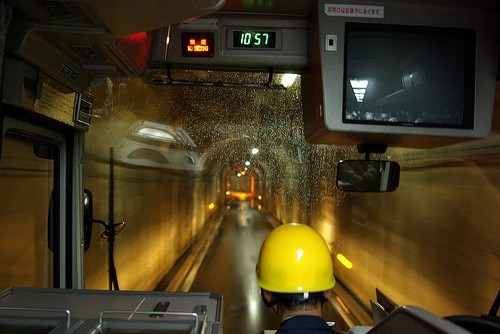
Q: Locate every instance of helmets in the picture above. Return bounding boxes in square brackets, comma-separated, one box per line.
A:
[255, 223, 337, 292]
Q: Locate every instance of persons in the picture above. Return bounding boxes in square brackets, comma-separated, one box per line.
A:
[255, 220, 341, 333]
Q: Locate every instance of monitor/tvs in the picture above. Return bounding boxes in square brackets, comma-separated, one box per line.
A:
[341, 17, 478, 133]
